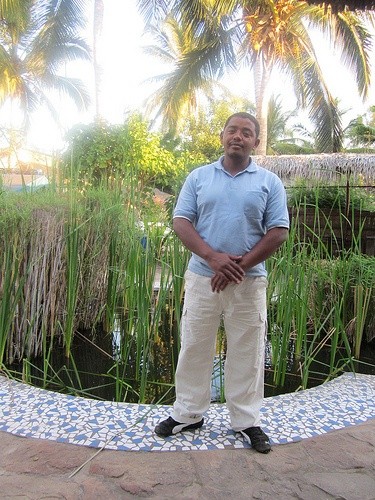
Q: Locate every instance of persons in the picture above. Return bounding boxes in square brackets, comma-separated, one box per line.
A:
[153, 112, 290, 453]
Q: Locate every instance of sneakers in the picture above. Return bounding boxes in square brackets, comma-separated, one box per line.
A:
[154, 417, 204, 436]
[238, 427, 271, 454]
